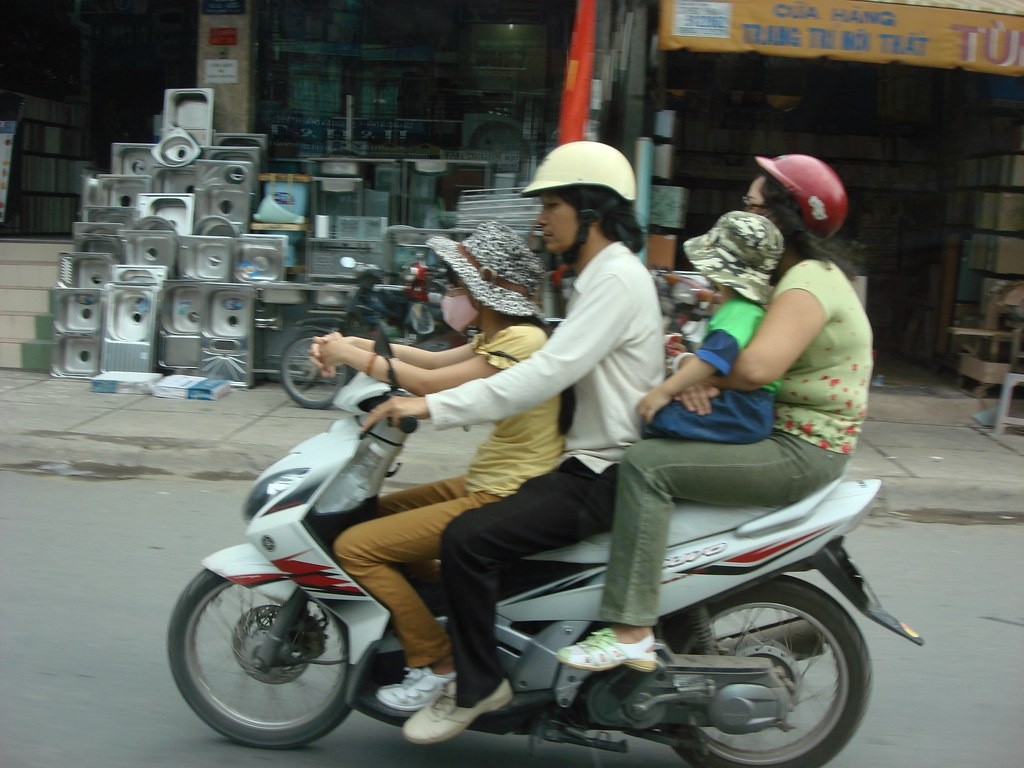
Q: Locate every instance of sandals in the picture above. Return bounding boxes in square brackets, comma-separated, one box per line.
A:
[557, 628, 657, 673]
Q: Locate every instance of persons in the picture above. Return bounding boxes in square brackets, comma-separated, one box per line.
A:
[559, 154, 873, 672]
[359, 142, 667, 743]
[310, 222, 576, 712]
[635, 211, 785, 444]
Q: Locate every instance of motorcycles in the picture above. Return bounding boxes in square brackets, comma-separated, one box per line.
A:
[278, 256, 452, 408]
[167, 315, 927, 768]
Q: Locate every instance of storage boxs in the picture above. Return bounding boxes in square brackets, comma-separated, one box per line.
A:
[661, 111, 1024, 293]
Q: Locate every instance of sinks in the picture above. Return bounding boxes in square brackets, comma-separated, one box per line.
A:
[51, 87, 546, 386]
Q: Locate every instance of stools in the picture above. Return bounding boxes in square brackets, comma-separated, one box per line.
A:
[992, 371, 1024, 443]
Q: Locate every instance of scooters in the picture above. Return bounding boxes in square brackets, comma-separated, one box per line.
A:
[401, 260, 562, 343]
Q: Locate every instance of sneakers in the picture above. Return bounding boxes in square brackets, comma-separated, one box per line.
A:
[376, 666, 457, 711]
[401, 677, 514, 745]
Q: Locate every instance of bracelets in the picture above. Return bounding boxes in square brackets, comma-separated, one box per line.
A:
[367, 354, 376, 376]
[672, 352, 694, 372]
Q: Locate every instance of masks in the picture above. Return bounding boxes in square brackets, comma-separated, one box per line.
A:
[442, 294, 488, 332]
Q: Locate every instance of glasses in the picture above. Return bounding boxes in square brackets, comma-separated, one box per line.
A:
[432, 279, 465, 292]
[742, 196, 771, 209]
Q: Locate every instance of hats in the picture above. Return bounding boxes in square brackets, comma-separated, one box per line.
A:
[683, 212, 786, 304]
[426, 221, 549, 326]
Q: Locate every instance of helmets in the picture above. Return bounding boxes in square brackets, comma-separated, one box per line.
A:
[521, 141, 636, 201]
[754, 154, 848, 240]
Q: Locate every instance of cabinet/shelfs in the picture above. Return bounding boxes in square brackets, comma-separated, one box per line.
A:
[0, 90, 96, 236]
